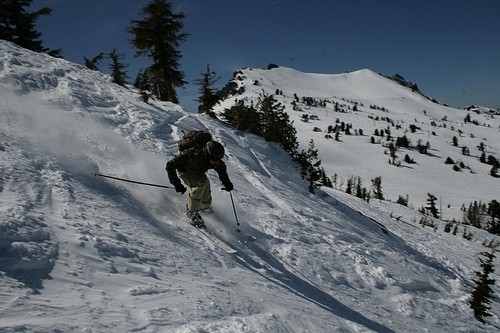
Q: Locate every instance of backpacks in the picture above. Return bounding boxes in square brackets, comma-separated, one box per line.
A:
[178, 130, 212, 173]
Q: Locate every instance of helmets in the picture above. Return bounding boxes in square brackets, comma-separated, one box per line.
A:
[202, 140, 224, 167]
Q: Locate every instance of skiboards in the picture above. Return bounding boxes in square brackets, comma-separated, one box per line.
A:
[172, 205, 256, 254]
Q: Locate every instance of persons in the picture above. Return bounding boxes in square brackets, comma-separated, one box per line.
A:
[166, 131, 233, 228]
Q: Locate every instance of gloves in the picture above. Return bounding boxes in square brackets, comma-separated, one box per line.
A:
[221, 179, 233, 191]
[174, 180, 187, 193]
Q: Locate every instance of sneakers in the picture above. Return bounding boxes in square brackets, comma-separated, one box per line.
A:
[200, 206, 213, 215]
[186, 210, 204, 225]
